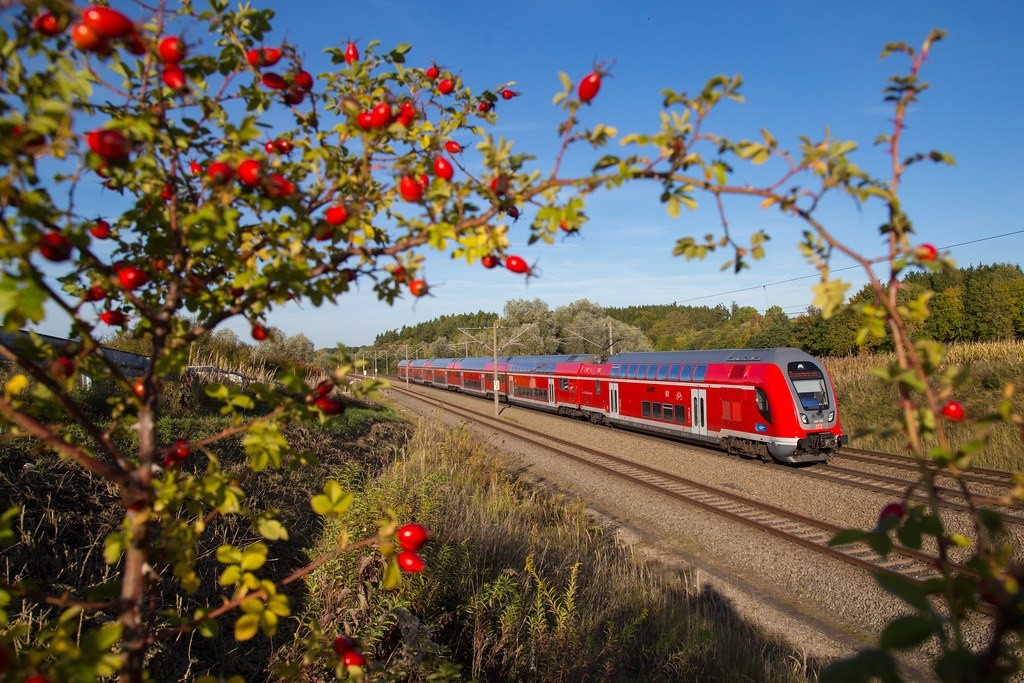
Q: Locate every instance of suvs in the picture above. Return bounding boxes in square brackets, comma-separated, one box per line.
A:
[196, 366, 214, 381]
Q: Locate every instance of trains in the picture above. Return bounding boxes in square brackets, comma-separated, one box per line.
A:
[397, 349, 844, 463]
